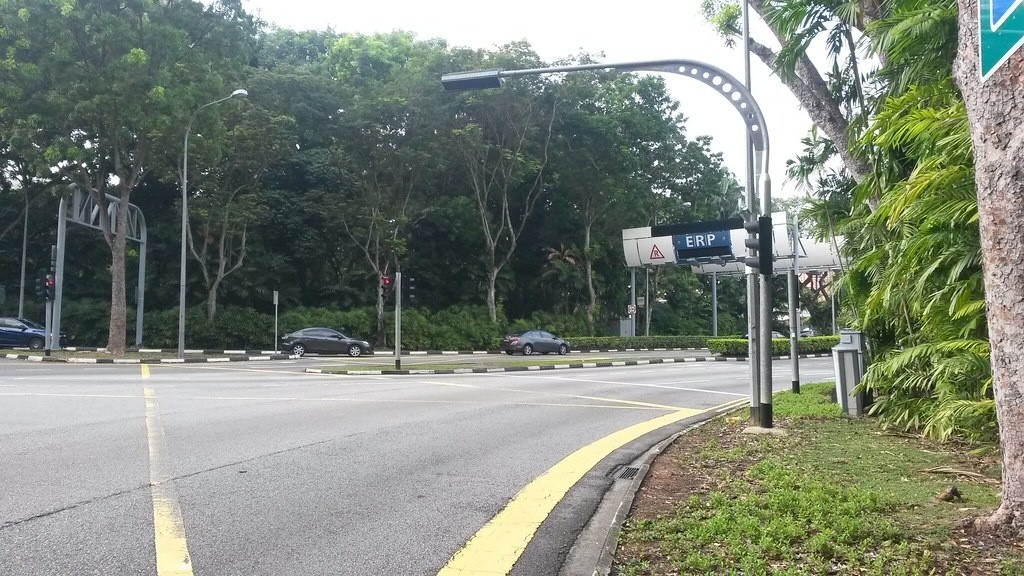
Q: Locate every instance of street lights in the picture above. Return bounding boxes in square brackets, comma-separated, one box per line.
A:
[645, 201, 693, 336]
[175, 87, 249, 359]
[9, 173, 29, 319]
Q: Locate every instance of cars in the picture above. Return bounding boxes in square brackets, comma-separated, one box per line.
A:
[0, 315, 70, 350]
[499, 328, 571, 356]
[281, 326, 373, 358]
[741, 330, 786, 339]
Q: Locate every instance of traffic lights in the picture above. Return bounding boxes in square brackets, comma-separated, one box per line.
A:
[35, 275, 45, 302]
[744, 214, 773, 275]
[382, 275, 396, 299]
[401, 275, 416, 304]
[45, 276, 55, 301]
